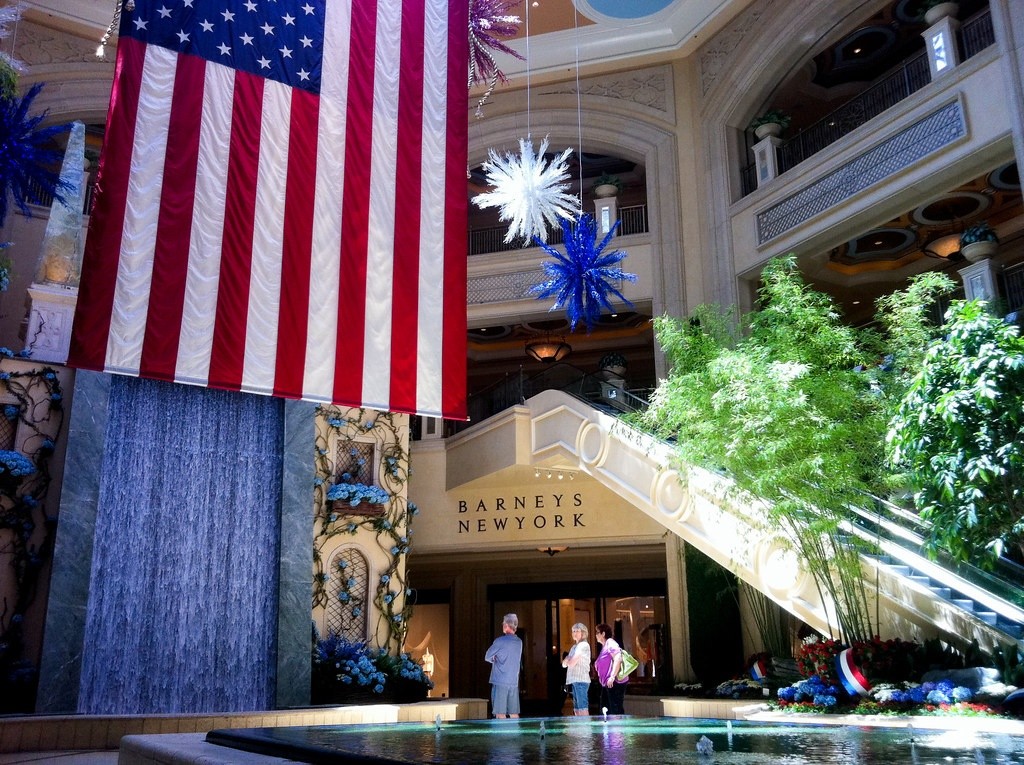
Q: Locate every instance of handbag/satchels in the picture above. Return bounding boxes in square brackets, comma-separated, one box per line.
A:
[615, 649, 639, 680]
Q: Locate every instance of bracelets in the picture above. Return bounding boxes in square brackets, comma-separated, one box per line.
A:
[610, 676, 614, 681]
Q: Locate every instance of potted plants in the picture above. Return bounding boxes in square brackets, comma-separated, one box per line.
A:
[591, 172, 625, 198]
[917, 0, 959, 26]
[750, 108, 792, 140]
[960, 220, 1000, 264]
[598, 351, 628, 381]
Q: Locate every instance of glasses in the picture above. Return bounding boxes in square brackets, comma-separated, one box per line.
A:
[596, 632, 600, 635]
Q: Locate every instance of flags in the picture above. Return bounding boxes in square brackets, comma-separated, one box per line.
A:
[64, 0, 471, 422]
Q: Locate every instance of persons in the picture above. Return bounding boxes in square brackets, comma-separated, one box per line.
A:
[562, 622, 591, 716]
[595, 623, 629, 715]
[422, 647, 434, 695]
[485, 612, 523, 718]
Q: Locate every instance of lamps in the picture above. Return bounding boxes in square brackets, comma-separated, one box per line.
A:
[524, 329, 573, 364]
[921, 217, 963, 263]
